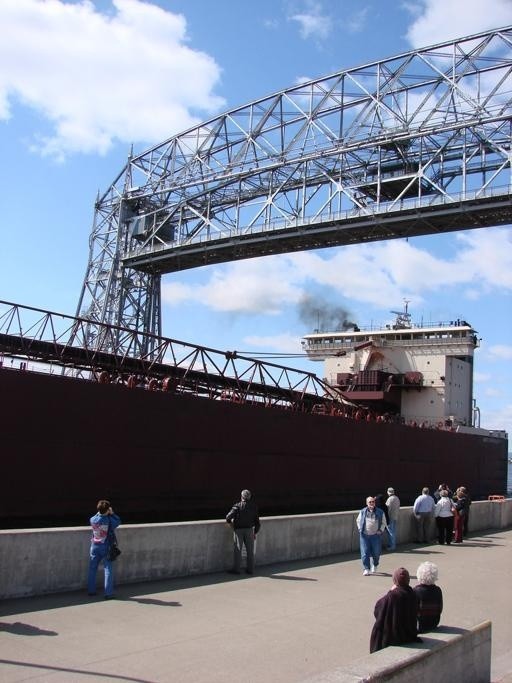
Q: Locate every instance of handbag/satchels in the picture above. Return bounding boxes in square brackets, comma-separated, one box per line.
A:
[451, 508, 457, 517]
[107, 545, 121, 562]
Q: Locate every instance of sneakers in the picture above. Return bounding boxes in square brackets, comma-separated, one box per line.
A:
[363, 565, 377, 576]
[228, 569, 253, 574]
[88, 592, 116, 599]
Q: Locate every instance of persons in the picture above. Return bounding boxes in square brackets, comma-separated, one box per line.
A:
[413, 483, 472, 545]
[226, 489, 261, 574]
[369, 561, 443, 654]
[87, 500, 121, 600]
[376, 487, 400, 552]
[356, 496, 386, 576]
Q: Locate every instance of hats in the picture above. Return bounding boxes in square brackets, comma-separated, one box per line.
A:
[393, 566, 410, 586]
[386, 487, 395, 495]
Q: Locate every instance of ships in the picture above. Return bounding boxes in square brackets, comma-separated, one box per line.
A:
[0, 301, 508, 529]
